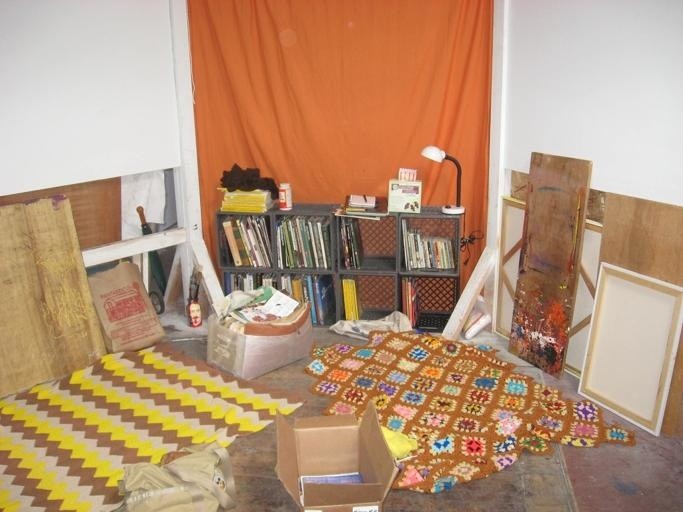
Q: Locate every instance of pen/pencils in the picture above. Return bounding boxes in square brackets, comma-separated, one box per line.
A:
[364, 195, 367, 202]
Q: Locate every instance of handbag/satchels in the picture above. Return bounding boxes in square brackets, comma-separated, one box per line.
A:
[120, 440, 227, 511]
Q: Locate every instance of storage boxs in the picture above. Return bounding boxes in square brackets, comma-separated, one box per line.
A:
[274, 401, 399, 512]
[207, 302, 315, 380]
[89, 261, 167, 354]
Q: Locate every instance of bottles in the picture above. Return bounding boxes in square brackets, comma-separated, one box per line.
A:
[184, 297, 201, 327]
[277, 183, 291, 210]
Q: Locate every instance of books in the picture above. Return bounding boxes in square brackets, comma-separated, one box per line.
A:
[217, 178, 455, 335]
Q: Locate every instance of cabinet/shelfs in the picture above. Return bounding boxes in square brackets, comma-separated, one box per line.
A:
[216, 203, 463, 332]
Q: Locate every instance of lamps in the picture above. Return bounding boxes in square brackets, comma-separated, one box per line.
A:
[420, 145, 465, 215]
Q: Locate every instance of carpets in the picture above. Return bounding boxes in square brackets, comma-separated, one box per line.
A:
[307, 330, 636, 494]
[0, 338, 306, 512]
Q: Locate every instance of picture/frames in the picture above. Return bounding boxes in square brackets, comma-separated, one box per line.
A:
[492, 196, 603, 380]
[577, 261, 682, 437]
